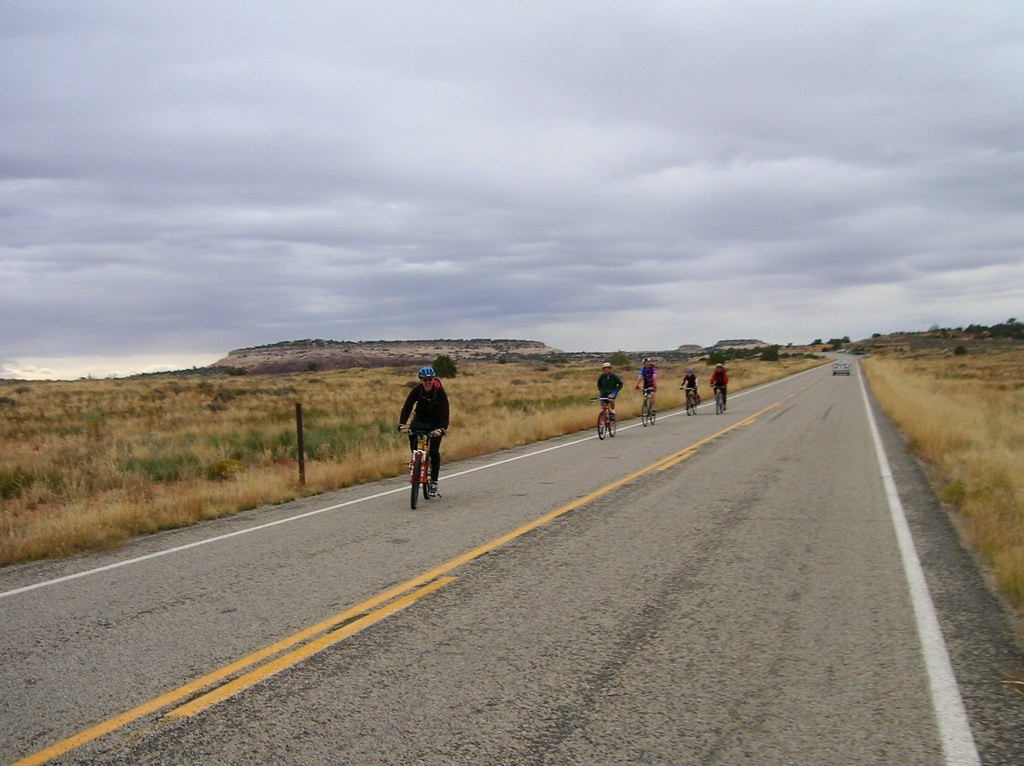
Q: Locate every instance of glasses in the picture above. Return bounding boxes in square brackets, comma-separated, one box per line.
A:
[422, 378, 432, 382]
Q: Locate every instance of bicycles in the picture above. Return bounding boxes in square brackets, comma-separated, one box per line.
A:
[711, 384, 726, 415]
[637, 386, 656, 427]
[680, 387, 701, 416]
[590, 396, 617, 440]
[396, 426, 442, 509]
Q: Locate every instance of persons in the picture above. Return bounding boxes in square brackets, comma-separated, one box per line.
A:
[680, 369, 697, 410]
[398, 366, 449, 497]
[635, 358, 658, 417]
[597, 361, 623, 428]
[709, 363, 729, 411]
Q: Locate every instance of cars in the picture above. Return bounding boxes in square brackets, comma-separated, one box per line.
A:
[831, 361, 851, 376]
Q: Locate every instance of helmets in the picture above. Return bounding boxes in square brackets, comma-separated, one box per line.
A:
[418, 367, 435, 379]
[716, 363, 722, 366]
[643, 357, 651, 362]
[603, 362, 612, 368]
[687, 368, 694, 373]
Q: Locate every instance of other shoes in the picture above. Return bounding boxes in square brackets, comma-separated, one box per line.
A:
[429, 485, 438, 497]
